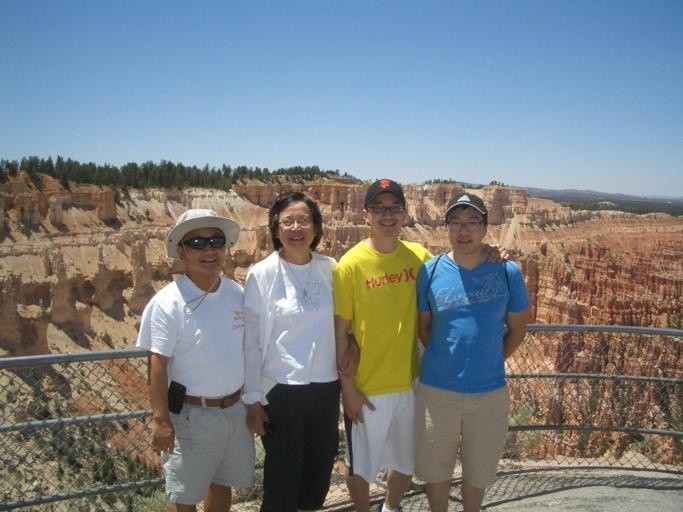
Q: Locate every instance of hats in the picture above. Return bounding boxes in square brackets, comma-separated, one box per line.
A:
[365, 179, 405, 206]
[444, 192, 487, 224]
[165, 209, 240, 258]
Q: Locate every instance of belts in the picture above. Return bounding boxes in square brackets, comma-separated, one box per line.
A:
[184, 389, 241, 409]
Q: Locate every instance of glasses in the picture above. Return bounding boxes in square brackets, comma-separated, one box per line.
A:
[180, 235, 225, 249]
[366, 204, 403, 214]
[280, 217, 311, 226]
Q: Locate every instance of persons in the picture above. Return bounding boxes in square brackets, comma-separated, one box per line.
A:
[134, 207, 256, 511]
[410, 191, 531, 511]
[237, 190, 362, 511]
[323, 177, 511, 510]
